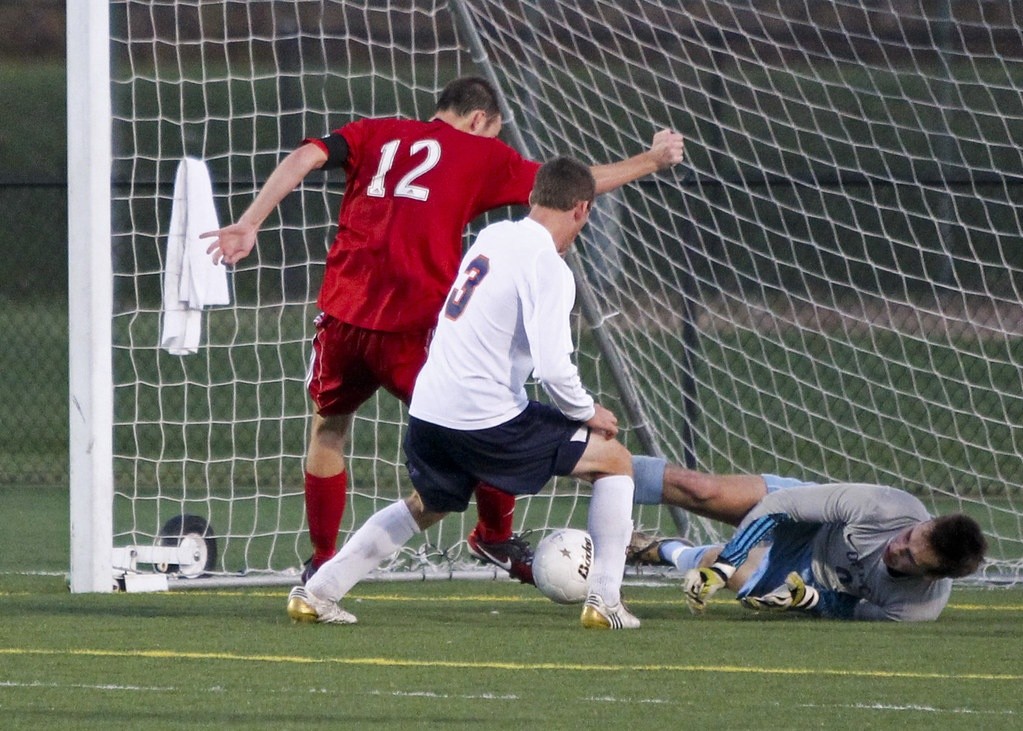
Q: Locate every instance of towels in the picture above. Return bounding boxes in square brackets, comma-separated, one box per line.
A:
[159, 155, 231, 357]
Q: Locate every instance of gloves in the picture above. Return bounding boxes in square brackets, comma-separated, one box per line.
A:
[685, 565, 729, 615]
[740, 571, 820, 610]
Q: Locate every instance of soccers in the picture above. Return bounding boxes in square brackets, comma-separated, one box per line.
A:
[531, 526, 596, 605]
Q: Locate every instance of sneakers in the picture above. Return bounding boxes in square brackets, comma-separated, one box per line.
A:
[580, 593, 641, 630]
[301, 555, 338, 584]
[625, 531, 694, 567]
[287, 586, 357, 624]
[467, 523, 537, 589]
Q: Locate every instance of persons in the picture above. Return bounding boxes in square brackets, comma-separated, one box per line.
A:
[199, 77, 685, 630]
[627, 454, 988, 622]
